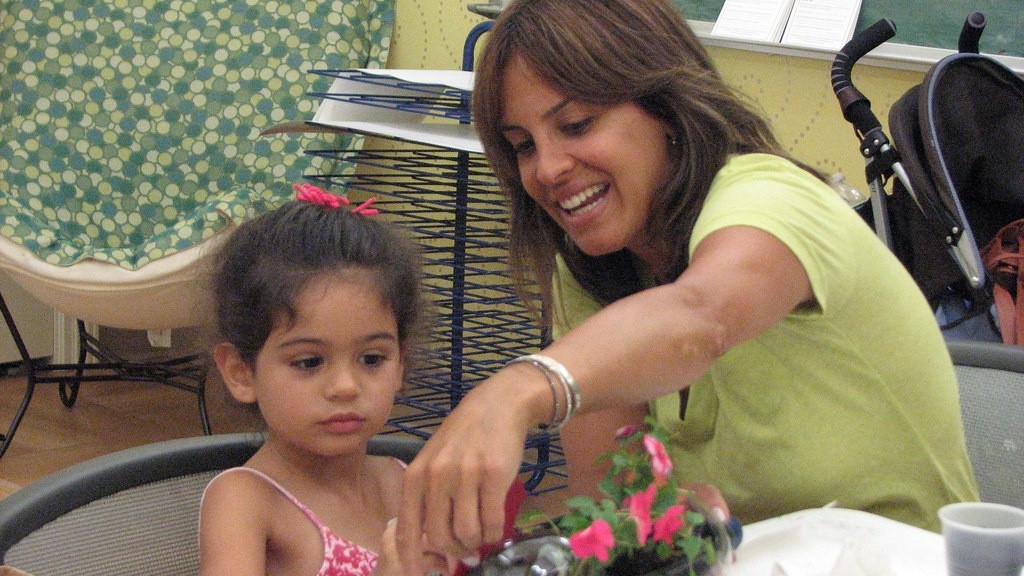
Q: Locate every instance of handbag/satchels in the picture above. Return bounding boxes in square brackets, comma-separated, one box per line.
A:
[977, 219, 1024, 347]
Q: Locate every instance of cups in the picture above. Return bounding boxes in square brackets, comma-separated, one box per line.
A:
[937, 502, 1024, 576]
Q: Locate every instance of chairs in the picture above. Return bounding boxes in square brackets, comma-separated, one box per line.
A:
[0, 0, 394, 459]
[946, 341, 1024, 510]
[0, 433, 429, 576]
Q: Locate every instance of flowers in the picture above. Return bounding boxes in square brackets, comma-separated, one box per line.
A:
[560, 413, 733, 576]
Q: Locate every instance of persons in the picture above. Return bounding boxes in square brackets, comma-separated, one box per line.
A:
[198, 183, 458, 576]
[395, 0, 981, 575]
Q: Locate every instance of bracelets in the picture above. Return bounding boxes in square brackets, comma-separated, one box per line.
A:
[503, 354, 582, 437]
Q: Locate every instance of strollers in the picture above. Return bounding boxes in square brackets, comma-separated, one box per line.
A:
[828, 12, 1023, 372]
